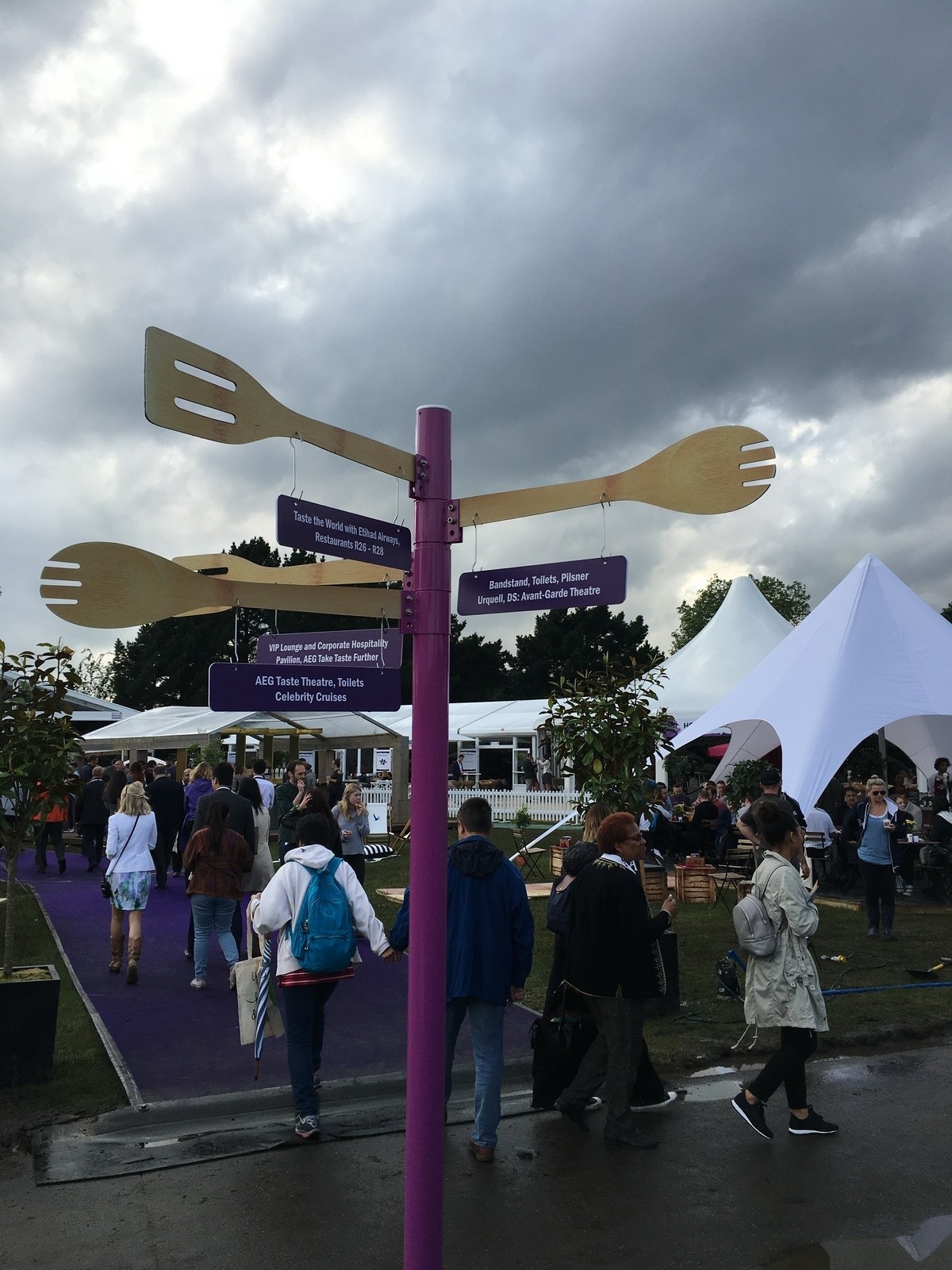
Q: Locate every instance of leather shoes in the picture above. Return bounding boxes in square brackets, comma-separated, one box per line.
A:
[155, 885, 165, 889]
[173, 872, 179, 876]
[617, 1129, 658, 1148]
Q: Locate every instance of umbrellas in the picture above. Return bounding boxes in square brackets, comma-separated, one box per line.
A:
[254, 932, 272, 1081]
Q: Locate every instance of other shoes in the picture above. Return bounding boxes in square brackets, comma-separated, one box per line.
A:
[87, 861, 97, 872]
[468, 1139, 495, 1160]
[59, 859, 67, 873]
[650, 850, 664, 861]
[184, 949, 192, 963]
[654, 857, 661, 865]
[109, 961, 120, 971]
[36, 868, 45, 873]
[884, 930, 899, 941]
[868, 928, 880, 936]
[555, 1097, 602, 1112]
[127, 960, 138, 983]
[631, 1091, 677, 1112]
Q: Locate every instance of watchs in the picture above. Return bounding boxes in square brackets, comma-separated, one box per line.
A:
[294, 804, 301, 812]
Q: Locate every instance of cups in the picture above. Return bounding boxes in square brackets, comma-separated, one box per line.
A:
[913, 835, 919, 842]
[883, 819, 891, 828]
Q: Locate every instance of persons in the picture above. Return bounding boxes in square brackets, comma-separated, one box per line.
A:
[522, 753, 952, 1110]
[851, 773, 903, 942]
[32, 742, 393, 988]
[250, 813, 392, 1136]
[385, 796, 535, 1160]
[451, 754, 465, 790]
[729, 801, 839, 1137]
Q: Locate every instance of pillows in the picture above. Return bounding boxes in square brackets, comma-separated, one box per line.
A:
[364, 844, 394, 858]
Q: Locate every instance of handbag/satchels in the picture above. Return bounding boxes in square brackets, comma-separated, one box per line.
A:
[530, 1004, 602, 1110]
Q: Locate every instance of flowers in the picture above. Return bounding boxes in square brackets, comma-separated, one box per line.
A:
[906, 818, 916, 833]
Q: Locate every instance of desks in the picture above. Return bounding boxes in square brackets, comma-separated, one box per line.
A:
[898, 841, 940, 846]
[674, 864, 716, 903]
[549, 845, 568, 876]
[644, 863, 668, 901]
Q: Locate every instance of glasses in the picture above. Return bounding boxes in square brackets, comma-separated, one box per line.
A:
[620, 833, 643, 842]
[868, 790, 885, 796]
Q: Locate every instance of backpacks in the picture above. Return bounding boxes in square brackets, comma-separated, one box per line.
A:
[285, 857, 356, 973]
[733, 864, 791, 956]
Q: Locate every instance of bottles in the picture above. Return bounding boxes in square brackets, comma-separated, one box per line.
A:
[275, 768, 287, 778]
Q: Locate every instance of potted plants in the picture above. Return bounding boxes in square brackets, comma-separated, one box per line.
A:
[535, 645, 693, 1019]
[511, 803, 533, 867]
[0, 639, 86, 1071]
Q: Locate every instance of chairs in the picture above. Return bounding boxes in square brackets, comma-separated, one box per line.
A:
[363, 802, 394, 844]
[451, 779, 500, 790]
[388, 817, 411, 853]
[643, 814, 952, 908]
[511, 828, 547, 880]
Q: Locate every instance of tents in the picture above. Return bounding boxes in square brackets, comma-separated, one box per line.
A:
[612, 577, 794, 712]
[509, 553, 952, 863]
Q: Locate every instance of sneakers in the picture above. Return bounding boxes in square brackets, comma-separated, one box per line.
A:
[229, 971, 238, 990]
[731, 1091, 774, 1139]
[312, 1072, 322, 1088]
[295, 1113, 321, 1137]
[190, 977, 205, 988]
[788, 1105, 838, 1134]
